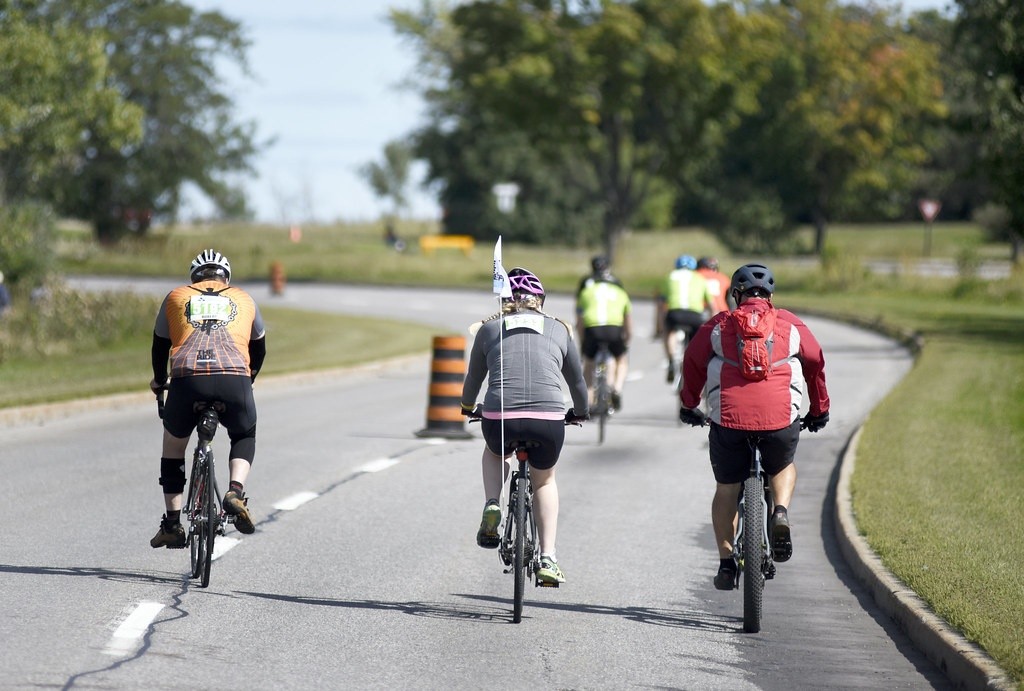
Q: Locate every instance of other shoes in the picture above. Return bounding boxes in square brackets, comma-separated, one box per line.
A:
[668, 365, 678, 382]
[611, 391, 622, 411]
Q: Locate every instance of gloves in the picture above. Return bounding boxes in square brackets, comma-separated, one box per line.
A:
[679, 406, 707, 428]
[802, 409, 830, 434]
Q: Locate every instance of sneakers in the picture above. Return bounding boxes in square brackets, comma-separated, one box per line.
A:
[150, 515, 187, 548]
[223, 491, 255, 533]
[537, 557, 566, 582]
[714, 566, 737, 590]
[478, 504, 501, 549]
[769, 510, 793, 562]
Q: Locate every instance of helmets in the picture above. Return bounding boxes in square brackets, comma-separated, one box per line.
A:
[593, 256, 610, 274]
[698, 257, 719, 271]
[189, 248, 232, 285]
[730, 264, 774, 295]
[501, 266, 546, 308]
[676, 255, 697, 269]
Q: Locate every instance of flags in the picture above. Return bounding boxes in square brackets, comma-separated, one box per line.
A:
[494, 237, 513, 298]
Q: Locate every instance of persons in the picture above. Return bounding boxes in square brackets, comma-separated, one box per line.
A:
[149, 249, 266, 548]
[653, 254, 720, 382]
[575, 253, 624, 296]
[577, 270, 632, 410]
[696, 257, 731, 314]
[679, 264, 830, 591]
[460, 266, 590, 583]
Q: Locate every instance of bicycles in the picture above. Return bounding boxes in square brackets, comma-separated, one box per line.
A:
[155, 381, 239, 586]
[462, 403, 589, 623]
[685, 410, 826, 633]
[592, 343, 614, 442]
